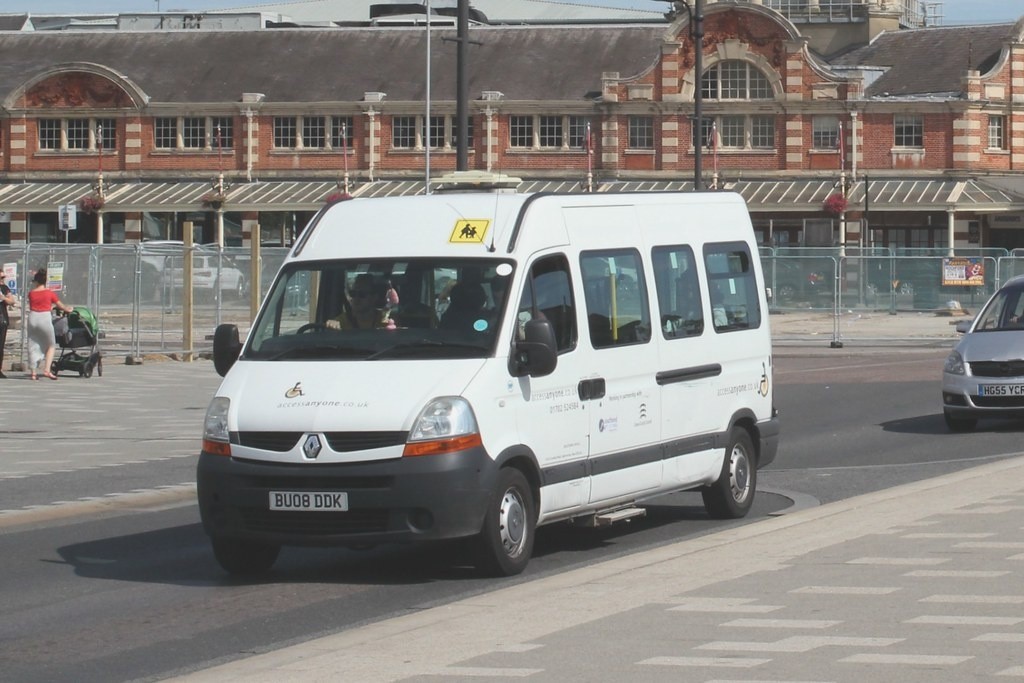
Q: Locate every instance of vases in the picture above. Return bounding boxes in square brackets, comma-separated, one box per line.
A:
[213, 201, 221, 209]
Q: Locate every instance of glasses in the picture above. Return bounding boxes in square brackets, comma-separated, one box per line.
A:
[0, 276, 5, 279]
[32, 279, 35, 282]
[345, 286, 370, 297]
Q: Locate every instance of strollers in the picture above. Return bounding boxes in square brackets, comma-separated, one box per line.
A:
[47, 304, 104, 378]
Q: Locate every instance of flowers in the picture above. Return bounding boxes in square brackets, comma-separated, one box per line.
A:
[82, 195, 104, 215]
[824, 194, 848, 215]
[202, 194, 226, 205]
[327, 193, 351, 202]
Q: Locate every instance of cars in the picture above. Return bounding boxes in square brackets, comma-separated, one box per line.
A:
[940, 275, 1024, 434]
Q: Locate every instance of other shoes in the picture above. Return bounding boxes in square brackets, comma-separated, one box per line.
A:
[0, 371, 8, 379]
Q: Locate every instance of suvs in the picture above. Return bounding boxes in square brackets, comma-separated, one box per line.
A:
[233, 246, 308, 308]
[159, 246, 246, 304]
[0, 241, 198, 305]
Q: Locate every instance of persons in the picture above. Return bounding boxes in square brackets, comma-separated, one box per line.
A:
[488, 274, 549, 341]
[28, 268, 73, 380]
[327, 272, 398, 331]
[0, 269, 16, 378]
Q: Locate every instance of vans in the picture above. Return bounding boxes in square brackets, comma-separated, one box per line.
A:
[197, 187, 780, 576]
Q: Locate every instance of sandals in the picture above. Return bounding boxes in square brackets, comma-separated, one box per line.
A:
[31, 374, 39, 379]
[43, 371, 57, 380]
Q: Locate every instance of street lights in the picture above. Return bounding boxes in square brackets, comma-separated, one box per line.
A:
[663, 0, 705, 192]
